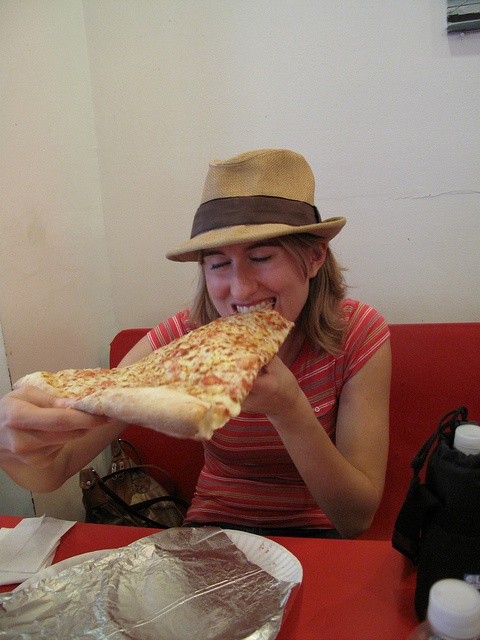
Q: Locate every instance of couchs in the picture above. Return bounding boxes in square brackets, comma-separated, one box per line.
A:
[110, 322, 479, 541]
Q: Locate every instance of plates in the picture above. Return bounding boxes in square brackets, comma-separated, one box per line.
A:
[222, 529, 304, 584]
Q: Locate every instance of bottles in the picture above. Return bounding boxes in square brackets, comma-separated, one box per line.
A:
[406, 578, 480, 639]
[452, 423, 479, 456]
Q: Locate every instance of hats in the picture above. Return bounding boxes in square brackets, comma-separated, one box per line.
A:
[166, 149, 346, 262]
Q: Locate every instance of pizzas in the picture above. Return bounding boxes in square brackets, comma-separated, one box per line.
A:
[13, 308, 296, 442]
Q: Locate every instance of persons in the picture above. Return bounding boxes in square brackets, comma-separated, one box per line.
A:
[1, 146, 393, 540]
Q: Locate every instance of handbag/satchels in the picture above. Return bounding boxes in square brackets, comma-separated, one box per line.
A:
[79, 437, 191, 529]
[390, 406, 480, 623]
